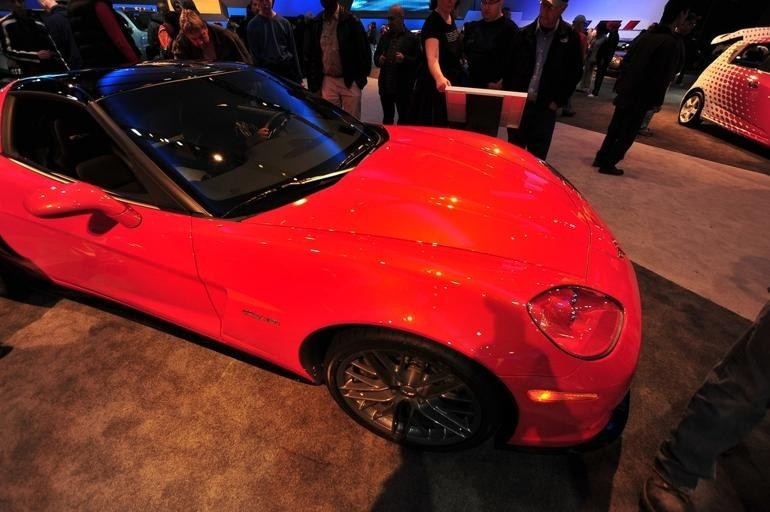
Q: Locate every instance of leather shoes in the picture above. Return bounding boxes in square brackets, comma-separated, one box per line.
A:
[593, 161, 600, 167]
[599, 169, 624, 175]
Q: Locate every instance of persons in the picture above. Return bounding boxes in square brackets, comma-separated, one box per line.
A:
[592, 1, 701, 175]
[146, 1, 373, 122]
[370, 0, 619, 158]
[643, 286, 769, 512]
[0, 1, 122, 80]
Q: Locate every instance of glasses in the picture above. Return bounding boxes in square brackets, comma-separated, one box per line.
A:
[539, 1, 554, 10]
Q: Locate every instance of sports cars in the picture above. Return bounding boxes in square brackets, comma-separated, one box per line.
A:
[0, 61, 644, 450]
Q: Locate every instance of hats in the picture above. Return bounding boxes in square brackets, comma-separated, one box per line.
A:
[547, 0, 567, 9]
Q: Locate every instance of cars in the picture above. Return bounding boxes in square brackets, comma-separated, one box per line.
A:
[113, 7, 151, 58]
[604, 38, 634, 70]
[677, 27, 770, 147]
[0, 9, 73, 83]
